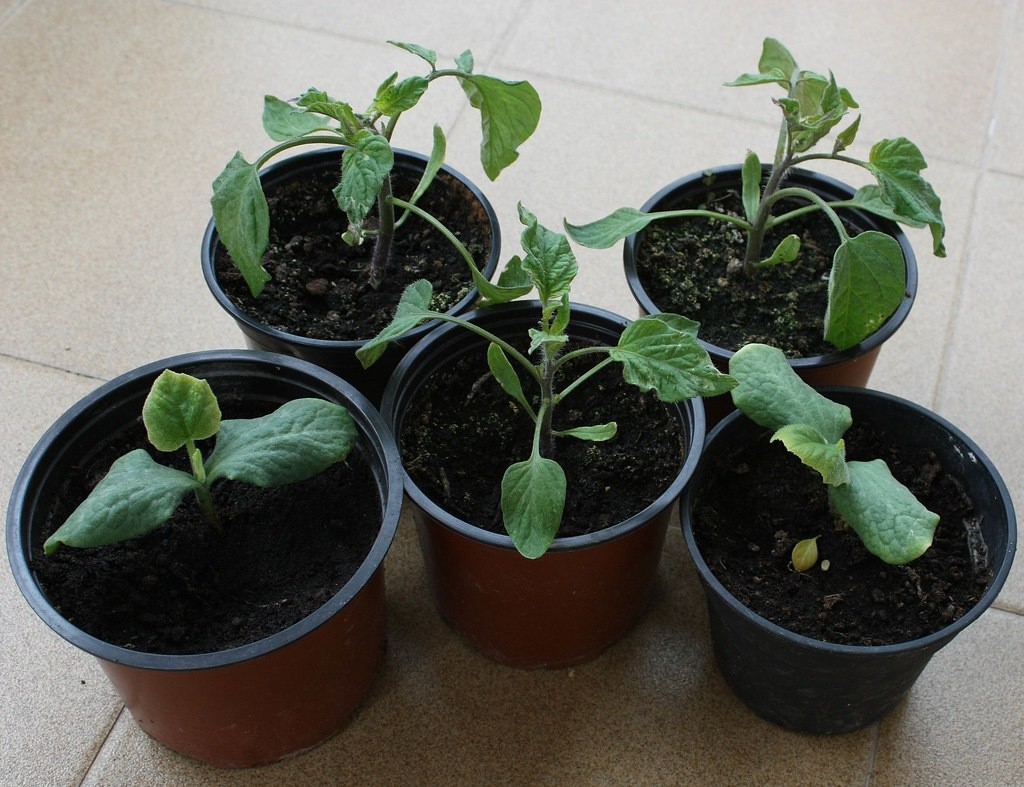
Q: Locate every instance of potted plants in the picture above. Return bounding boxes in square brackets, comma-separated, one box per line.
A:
[7, 37, 1017, 768]
